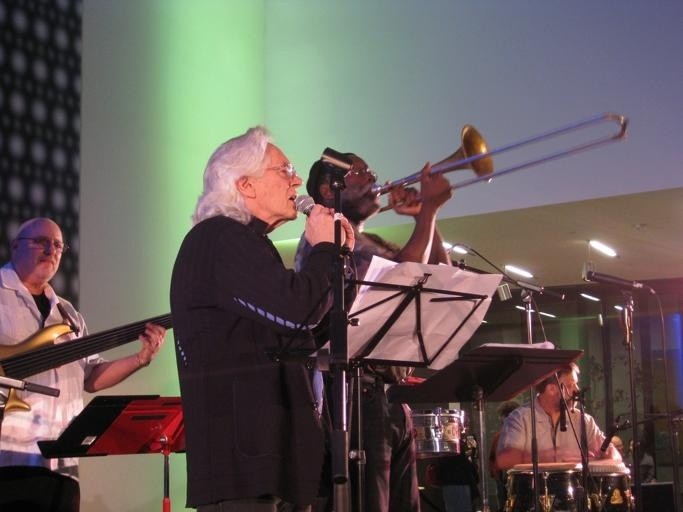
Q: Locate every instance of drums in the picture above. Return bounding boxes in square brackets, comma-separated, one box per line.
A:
[510, 462, 637, 512]
[411, 413, 465, 460]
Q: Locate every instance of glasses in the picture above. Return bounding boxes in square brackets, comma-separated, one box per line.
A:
[353, 168, 378, 182]
[14, 237, 69, 254]
[266, 164, 295, 180]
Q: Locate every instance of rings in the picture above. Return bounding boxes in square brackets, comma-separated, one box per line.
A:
[319, 207, 331, 215]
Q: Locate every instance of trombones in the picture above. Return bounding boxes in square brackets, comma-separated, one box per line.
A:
[370, 112, 628, 212]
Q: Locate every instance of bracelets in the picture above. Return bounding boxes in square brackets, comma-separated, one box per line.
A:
[136, 352, 140, 367]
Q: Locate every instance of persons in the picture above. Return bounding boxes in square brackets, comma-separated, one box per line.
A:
[487, 397, 522, 512]
[291, 152, 454, 511]
[495, 359, 623, 471]
[606, 435, 624, 459]
[0, 216, 168, 512]
[167, 124, 355, 510]
[628, 434, 658, 483]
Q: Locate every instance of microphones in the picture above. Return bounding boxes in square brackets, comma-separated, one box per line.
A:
[559, 384, 567, 432]
[583, 271, 655, 294]
[294, 194, 315, 218]
[601, 417, 619, 452]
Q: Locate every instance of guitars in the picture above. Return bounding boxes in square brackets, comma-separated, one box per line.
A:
[0, 312, 175, 413]
